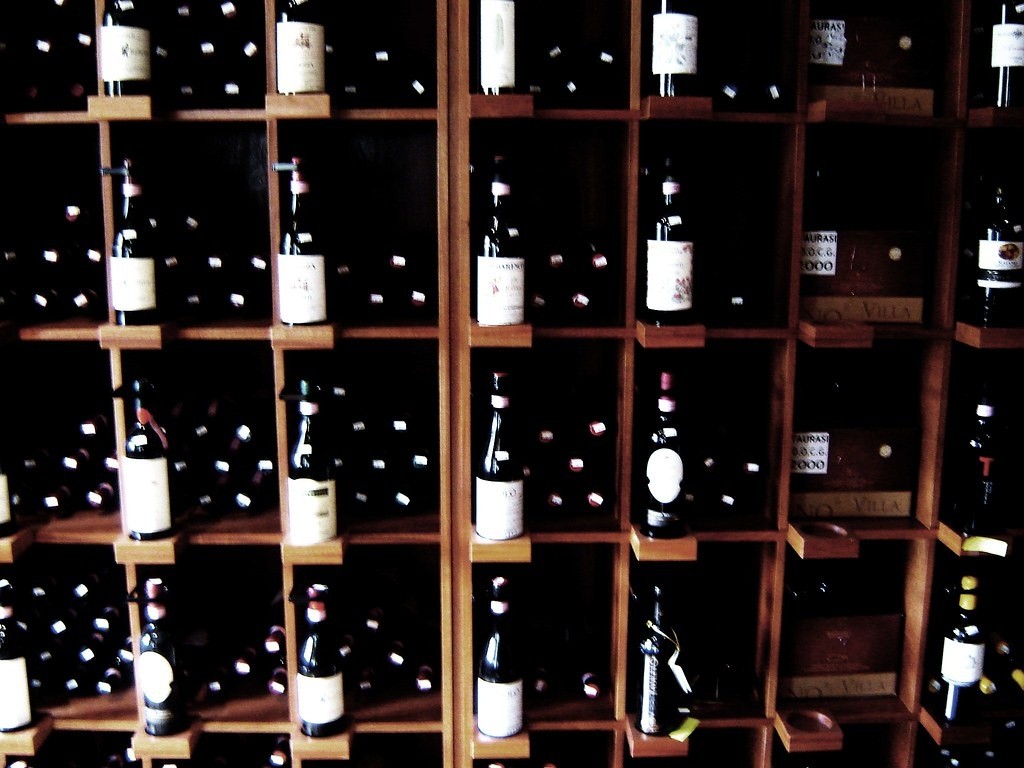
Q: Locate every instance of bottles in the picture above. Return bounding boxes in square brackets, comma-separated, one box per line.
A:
[0, 0, 1024, 768]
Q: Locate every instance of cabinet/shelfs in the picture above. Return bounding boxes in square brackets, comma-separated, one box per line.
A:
[0, 0, 1024, 768]
